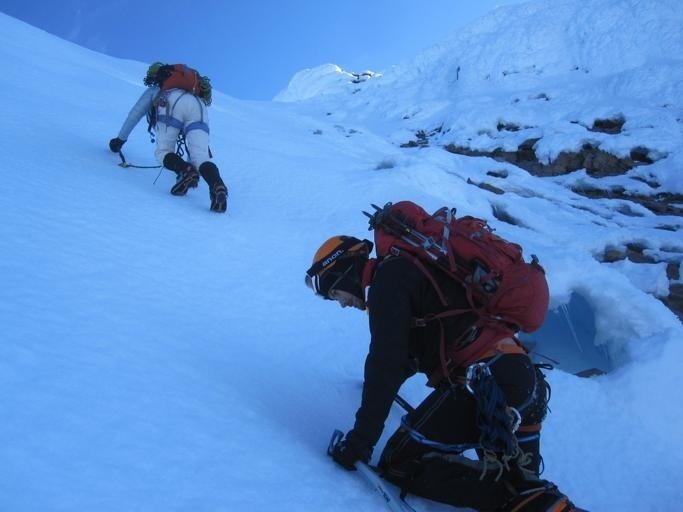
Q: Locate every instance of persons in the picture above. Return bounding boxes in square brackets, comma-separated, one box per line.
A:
[304, 235, 584, 511]
[108, 87, 229, 213]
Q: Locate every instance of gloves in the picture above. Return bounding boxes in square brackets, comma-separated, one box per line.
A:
[110, 137, 127, 152]
[333, 429, 373, 468]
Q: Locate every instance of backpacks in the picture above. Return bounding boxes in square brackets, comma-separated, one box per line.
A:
[157, 64, 201, 96]
[374, 201, 550, 333]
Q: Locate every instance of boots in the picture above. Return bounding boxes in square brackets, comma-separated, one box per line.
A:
[163, 153, 227, 213]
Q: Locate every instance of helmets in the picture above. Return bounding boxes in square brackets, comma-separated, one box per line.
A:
[147, 62, 164, 76]
[306, 235, 373, 300]
